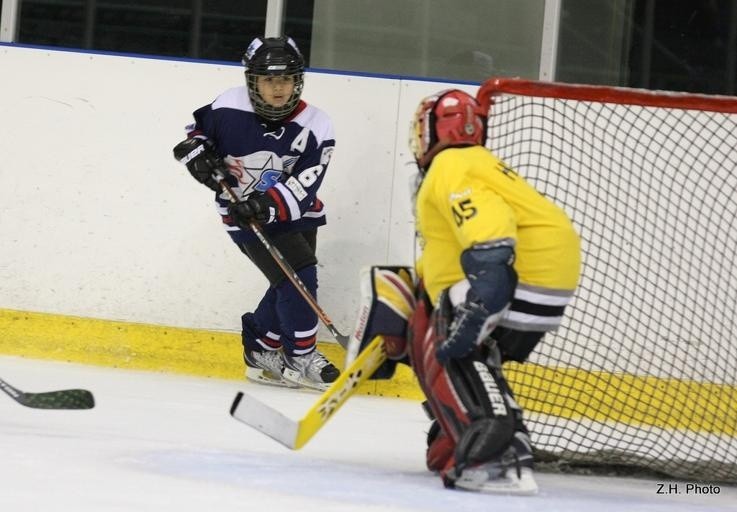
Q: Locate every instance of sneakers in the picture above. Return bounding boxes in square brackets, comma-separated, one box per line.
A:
[243, 343, 285, 371]
[445, 430, 538, 471]
[281, 344, 340, 382]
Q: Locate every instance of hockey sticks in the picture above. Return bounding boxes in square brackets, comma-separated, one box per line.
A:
[228, 335, 407, 450]
[211, 169, 348, 350]
[0, 380, 94, 409]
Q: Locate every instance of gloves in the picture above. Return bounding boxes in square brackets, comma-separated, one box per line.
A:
[228, 190, 279, 228]
[436, 305, 510, 363]
[172, 134, 232, 192]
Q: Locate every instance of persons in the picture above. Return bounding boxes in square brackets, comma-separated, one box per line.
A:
[168, 35, 343, 390]
[345, 85, 583, 490]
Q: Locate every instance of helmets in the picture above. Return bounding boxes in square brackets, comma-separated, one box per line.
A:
[241, 35, 305, 121]
[409, 88, 485, 167]
